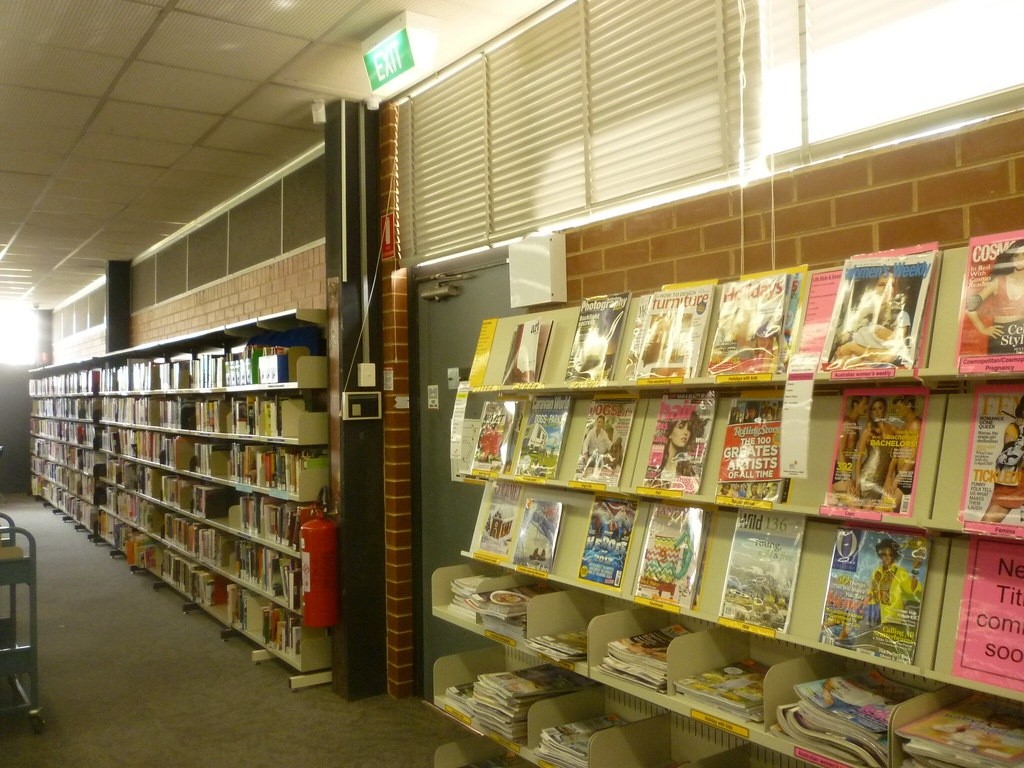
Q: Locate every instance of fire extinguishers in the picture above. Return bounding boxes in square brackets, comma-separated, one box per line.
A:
[300, 485, 339, 627]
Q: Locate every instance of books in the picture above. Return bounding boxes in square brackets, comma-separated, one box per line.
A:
[715, 397, 787, 504]
[27, 346, 323, 663]
[435, 482, 1023, 768]
[565, 290, 632, 380]
[501, 318, 555, 385]
[574, 399, 638, 488]
[470, 392, 574, 479]
[960, 237, 1024, 358]
[624, 250, 936, 380]
[963, 392, 1024, 527]
[644, 392, 718, 495]
[827, 393, 926, 515]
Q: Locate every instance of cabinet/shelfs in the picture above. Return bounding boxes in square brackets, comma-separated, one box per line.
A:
[99, 355, 332, 691]
[429, 248, 1024, 768]
[29, 391, 102, 540]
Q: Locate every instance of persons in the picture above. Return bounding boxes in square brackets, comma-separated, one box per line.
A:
[580, 414, 614, 466]
[660, 407, 709, 478]
[832, 269, 910, 368]
[981, 394, 1024, 523]
[829, 394, 923, 514]
[965, 239, 1024, 355]
[721, 399, 785, 503]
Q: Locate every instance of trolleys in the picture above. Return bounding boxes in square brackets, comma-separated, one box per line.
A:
[0, 511, 46, 735]
